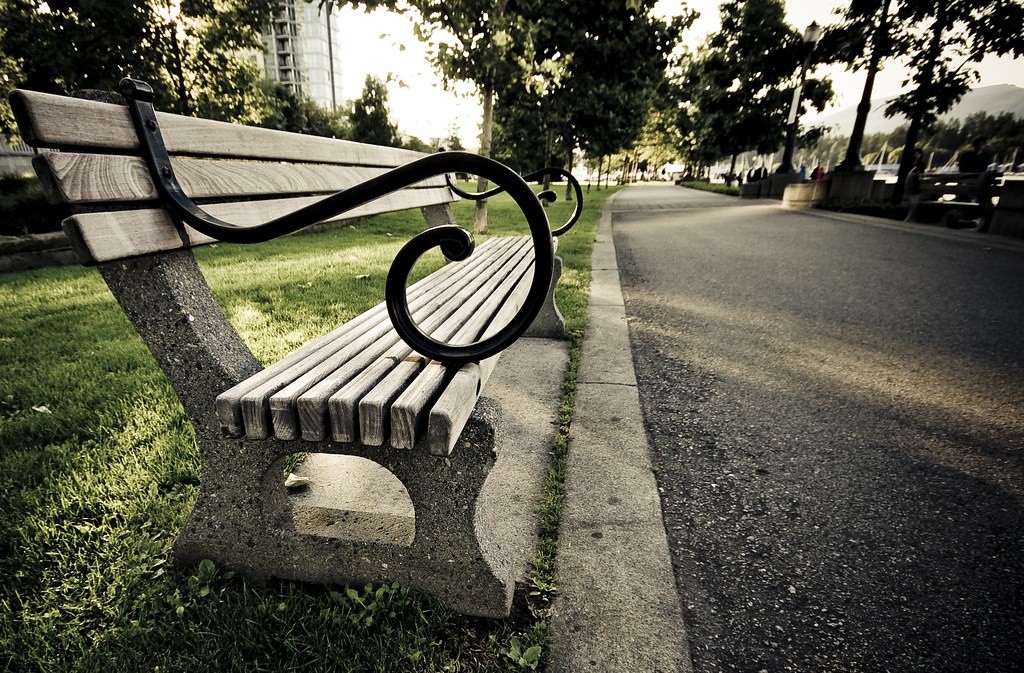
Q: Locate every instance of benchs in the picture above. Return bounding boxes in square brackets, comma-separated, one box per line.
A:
[903, 165, 1003, 233]
[10, 78, 583, 618]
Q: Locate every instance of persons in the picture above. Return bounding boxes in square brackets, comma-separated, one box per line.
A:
[662, 168, 666, 175]
[721, 169, 745, 186]
[957, 136, 988, 202]
[912, 148, 927, 173]
[811, 162, 825, 180]
[800, 164, 806, 175]
[747, 162, 768, 183]
